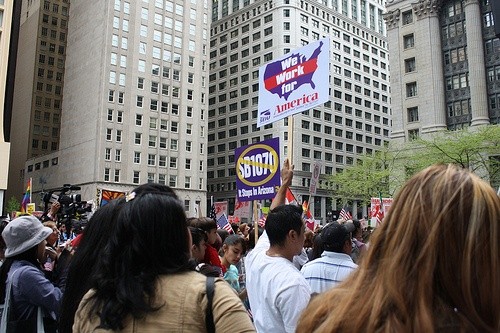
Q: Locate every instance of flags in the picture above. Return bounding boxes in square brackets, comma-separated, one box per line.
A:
[302, 201, 308, 214]
[217, 214, 231, 234]
[306, 212, 316, 230]
[258, 214, 266, 228]
[285, 188, 295, 202]
[376, 192, 384, 224]
[340, 204, 351, 220]
[21, 178, 30, 213]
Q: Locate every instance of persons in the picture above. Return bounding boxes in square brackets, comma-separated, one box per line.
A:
[0, 183, 257, 333]
[243, 156, 500, 333]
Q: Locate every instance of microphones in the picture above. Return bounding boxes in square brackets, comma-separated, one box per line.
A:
[69, 186, 81, 190]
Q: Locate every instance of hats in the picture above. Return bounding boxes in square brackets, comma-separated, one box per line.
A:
[2, 216, 53, 258]
[319, 218, 356, 246]
